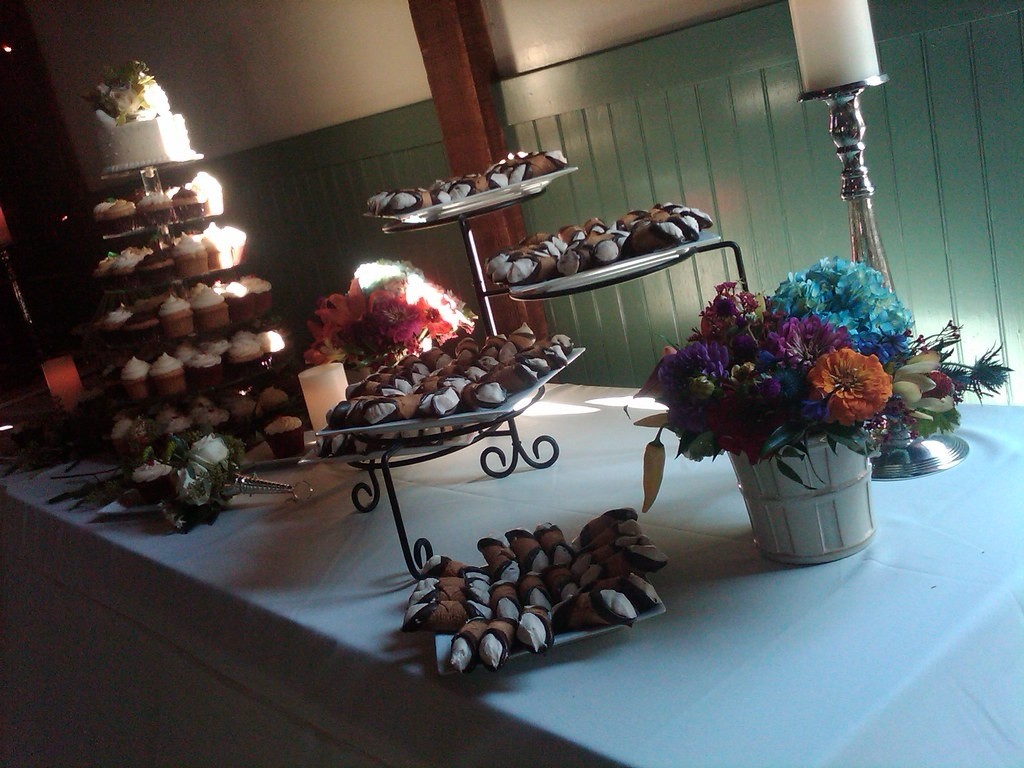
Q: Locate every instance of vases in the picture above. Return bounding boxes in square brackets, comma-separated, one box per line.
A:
[725, 435, 880, 564]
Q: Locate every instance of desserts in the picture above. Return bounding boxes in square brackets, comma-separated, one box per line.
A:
[483, 202, 713, 286]
[366, 147, 569, 214]
[315, 321, 573, 457]
[400, 507, 668, 677]
[10, 421, 33, 446]
[93, 170, 305, 461]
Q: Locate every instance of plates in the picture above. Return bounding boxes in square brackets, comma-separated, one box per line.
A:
[316, 347, 588, 437]
[363, 165, 579, 224]
[507, 230, 723, 296]
[434, 596, 668, 677]
[98, 489, 161, 515]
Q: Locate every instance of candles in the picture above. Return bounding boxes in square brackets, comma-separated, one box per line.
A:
[787, 0, 880, 93]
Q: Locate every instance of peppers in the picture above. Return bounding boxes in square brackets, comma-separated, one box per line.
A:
[642, 423, 669, 513]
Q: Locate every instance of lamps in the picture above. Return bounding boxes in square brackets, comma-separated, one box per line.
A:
[298, 362, 349, 440]
[40, 355, 82, 397]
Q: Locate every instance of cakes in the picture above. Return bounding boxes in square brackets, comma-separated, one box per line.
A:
[89, 58, 196, 172]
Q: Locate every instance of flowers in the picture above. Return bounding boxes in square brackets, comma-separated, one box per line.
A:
[80, 61, 171, 127]
[304, 257, 479, 375]
[66, 414, 245, 534]
[622, 254, 1015, 514]
[1, 387, 113, 477]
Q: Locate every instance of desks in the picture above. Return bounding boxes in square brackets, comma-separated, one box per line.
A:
[0, 382, 1024, 768]
